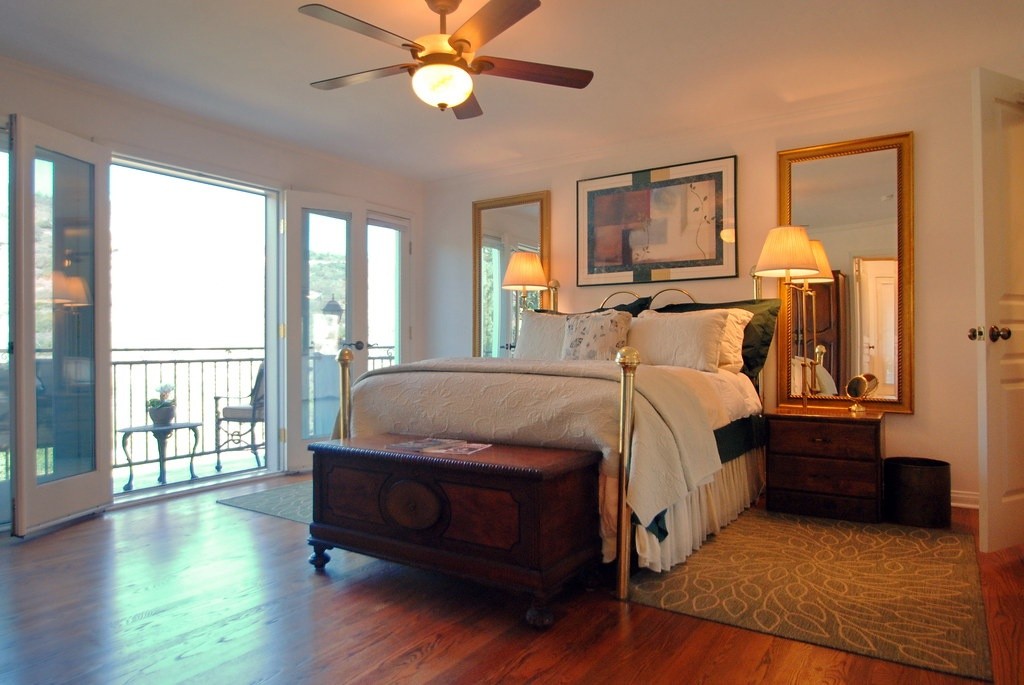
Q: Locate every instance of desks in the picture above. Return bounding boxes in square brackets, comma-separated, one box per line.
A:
[116, 421, 204, 492]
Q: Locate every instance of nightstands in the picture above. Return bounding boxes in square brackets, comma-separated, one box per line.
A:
[760, 409, 887, 526]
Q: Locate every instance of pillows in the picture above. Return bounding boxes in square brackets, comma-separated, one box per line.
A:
[651, 298, 782, 382]
[626, 310, 730, 374]
[534, 295, 652, 318]
[637, 308, 755, 375]
[512, 306, 634, 365]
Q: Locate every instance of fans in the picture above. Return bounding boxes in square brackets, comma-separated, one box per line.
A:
[295, 0, 594, 122]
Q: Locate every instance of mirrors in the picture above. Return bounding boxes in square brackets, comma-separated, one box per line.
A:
[774, 131, 918, 416]
[860, 373, 879, 397]
[845, 375, 868, 401]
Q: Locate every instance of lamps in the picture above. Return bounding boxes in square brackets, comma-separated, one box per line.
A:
[410, 33, 476, 113]
[471, 189, 553, 358]
[501, 250, 550, 312]
[787, 238, 836, 397]
[754, 225, 821, 407]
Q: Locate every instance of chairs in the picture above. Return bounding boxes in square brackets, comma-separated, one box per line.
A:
[213, 360, 266, 472]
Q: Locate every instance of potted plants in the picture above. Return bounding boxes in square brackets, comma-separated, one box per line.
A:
[146, 382, 179, 426]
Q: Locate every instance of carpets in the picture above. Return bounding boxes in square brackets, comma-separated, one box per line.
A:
[625, 511, 996, 684]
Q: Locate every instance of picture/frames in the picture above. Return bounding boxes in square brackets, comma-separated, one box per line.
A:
[575, 155, 740, 287]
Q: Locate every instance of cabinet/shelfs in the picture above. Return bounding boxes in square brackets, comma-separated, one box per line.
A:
[306, 432, 605, 633]
[792, 269, 849, 397]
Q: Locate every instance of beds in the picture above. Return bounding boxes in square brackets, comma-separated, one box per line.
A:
[349, 353, 766, 576]
